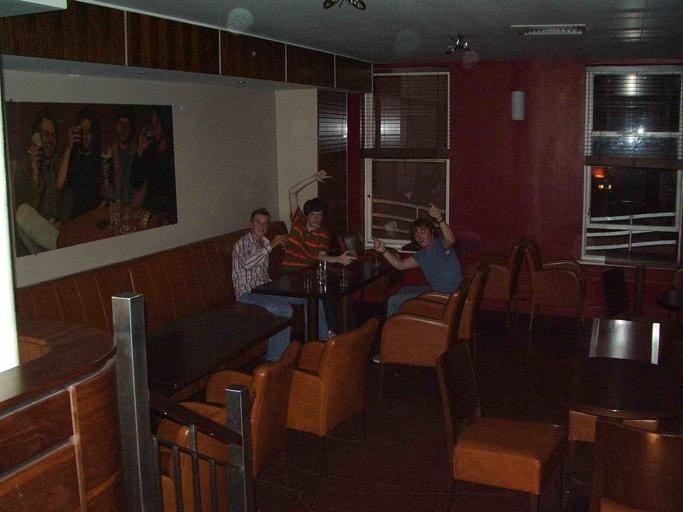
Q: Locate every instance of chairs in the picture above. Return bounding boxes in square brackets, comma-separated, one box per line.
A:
[463, 240, 521, 327]
[287, 317, 379, 437]
[523, 238, 585, 329]
[399, 269, 490, 373]
[160, 340, 300, 482]
[587, 315, 660, 365]
[376, 287, 468, 425]
[435, 339, 565, 510]
[566, 408, 682, 511]
[600, 266, 654, 323]
[161, 407, 231, 510]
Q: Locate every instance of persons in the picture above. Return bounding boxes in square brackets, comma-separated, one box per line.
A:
[230, 206, 338, 362]
[11, 106, 175, 256]
[279, 169, 358, 330]
[370, 202, 464, 366]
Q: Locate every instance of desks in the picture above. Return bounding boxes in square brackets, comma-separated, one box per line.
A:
[251, 254, 390, 341]
[534, 355, 680, 424]
[657, 286, 681, 315]
[144, 300, 293, 400]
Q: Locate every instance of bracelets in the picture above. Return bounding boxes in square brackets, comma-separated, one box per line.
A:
[436, 218, 443, 223]
[335, 256, 339, 263]
[380, 248, 386, 254]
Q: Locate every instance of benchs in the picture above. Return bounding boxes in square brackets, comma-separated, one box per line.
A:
[12, 221, 288, 336]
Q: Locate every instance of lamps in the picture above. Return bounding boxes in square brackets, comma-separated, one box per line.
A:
[511, 90, 525, 121]
